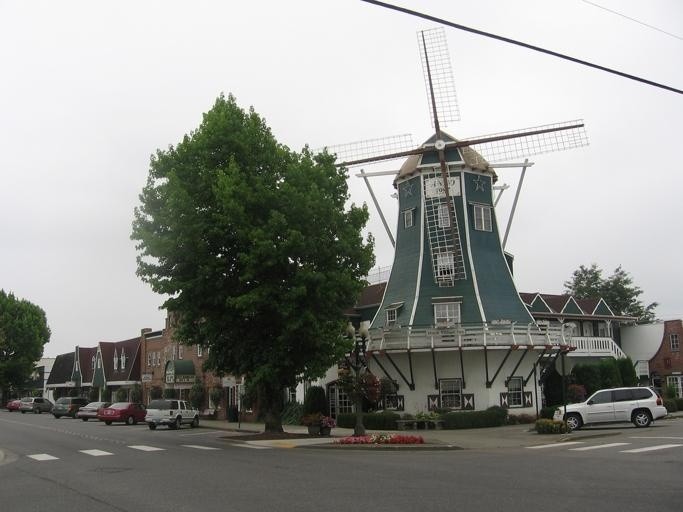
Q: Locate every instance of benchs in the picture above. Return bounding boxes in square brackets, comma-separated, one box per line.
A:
[395, 420, 445, 430]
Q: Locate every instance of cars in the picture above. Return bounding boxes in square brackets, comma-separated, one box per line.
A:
[5, 397, 199, 429]
[553, 385, 667, 431]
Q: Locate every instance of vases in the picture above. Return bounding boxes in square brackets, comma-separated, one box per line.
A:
[308, 425, 331, 437]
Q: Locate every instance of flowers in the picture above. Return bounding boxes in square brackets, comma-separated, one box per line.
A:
[300, 411, 335, 428]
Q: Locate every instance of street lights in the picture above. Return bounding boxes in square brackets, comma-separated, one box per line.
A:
[341, 321, 370, 437]
